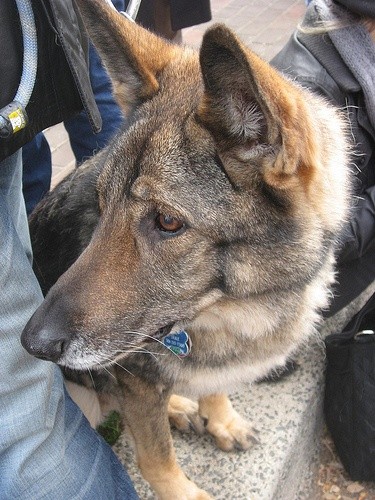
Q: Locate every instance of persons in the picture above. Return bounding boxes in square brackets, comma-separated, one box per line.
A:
[0, 2, 217, 500]
[268, 0, 374, 337]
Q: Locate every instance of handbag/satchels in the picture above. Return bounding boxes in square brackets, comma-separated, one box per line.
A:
[324, 288, 374, 485]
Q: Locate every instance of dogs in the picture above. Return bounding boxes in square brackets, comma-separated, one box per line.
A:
[20, 1, 366, 499]
